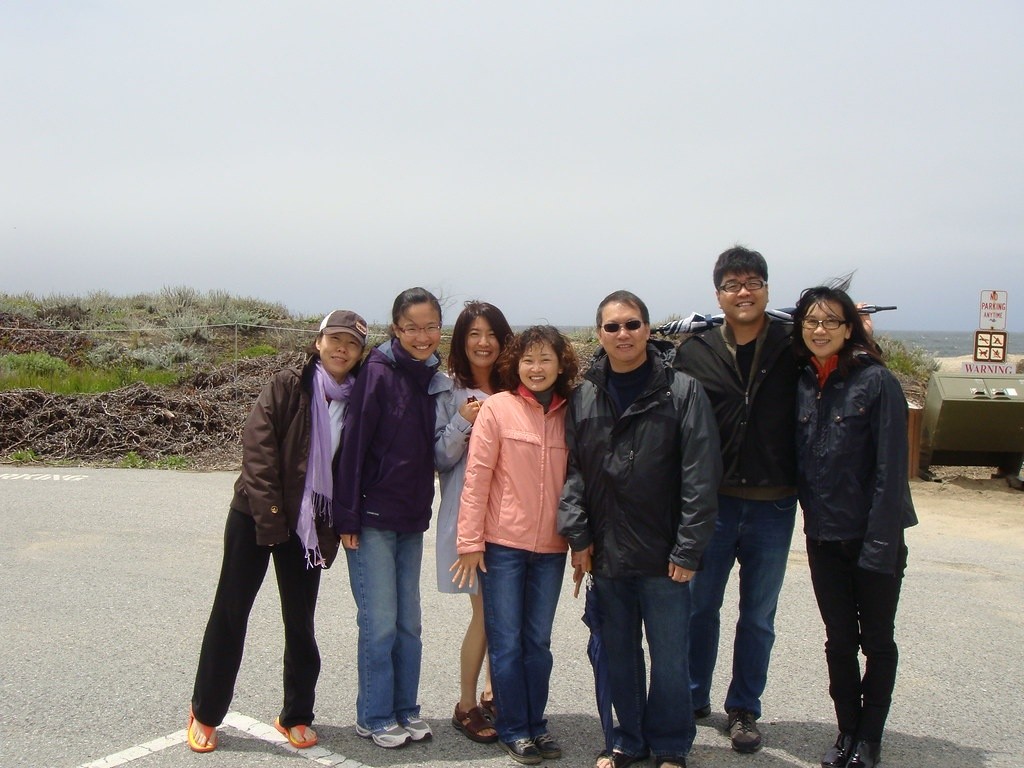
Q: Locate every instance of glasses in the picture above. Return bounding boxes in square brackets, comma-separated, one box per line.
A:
[799, 318, 848, 330]
[395, 324, 441, 336]
[599, 320, 644, 333]
[718, 279, 767, 293]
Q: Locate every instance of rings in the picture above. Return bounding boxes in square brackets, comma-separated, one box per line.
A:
[681, 574, 688, 578]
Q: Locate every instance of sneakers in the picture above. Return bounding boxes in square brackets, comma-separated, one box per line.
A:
[693, 703, 711, 720]
[402, 716, 433, 743]
[726, 709, 763, 752]
[356, 722, 410, 749]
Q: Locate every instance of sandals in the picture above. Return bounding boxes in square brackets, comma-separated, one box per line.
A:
[189, 705, 218, 753]
[596, 749, 640, 768]
[655, 755, 686, 768]
[451, 702, 498, 744]
[274, 714, 318, 749]
[478, 691, 497, 717]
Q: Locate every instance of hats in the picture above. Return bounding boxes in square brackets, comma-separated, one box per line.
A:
[319, 310, 367, 346]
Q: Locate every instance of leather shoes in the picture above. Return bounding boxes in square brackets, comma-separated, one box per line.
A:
[497, 738, 542, 764]
[823, 736, 881, 768]
[530, 732, 561, 759]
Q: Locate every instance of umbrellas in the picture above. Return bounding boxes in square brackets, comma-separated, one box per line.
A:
[581, 556, 614, 768]
[593, 304, 897, 361]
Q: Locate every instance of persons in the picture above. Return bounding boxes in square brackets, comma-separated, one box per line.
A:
[332, 287, 442, 749]
[672, 246, 873, 754]
[428, 302, 585, 764]
[187, 309, 368, 753]
[557, 290, 723, 768]
[795, 286, 919, 768]
